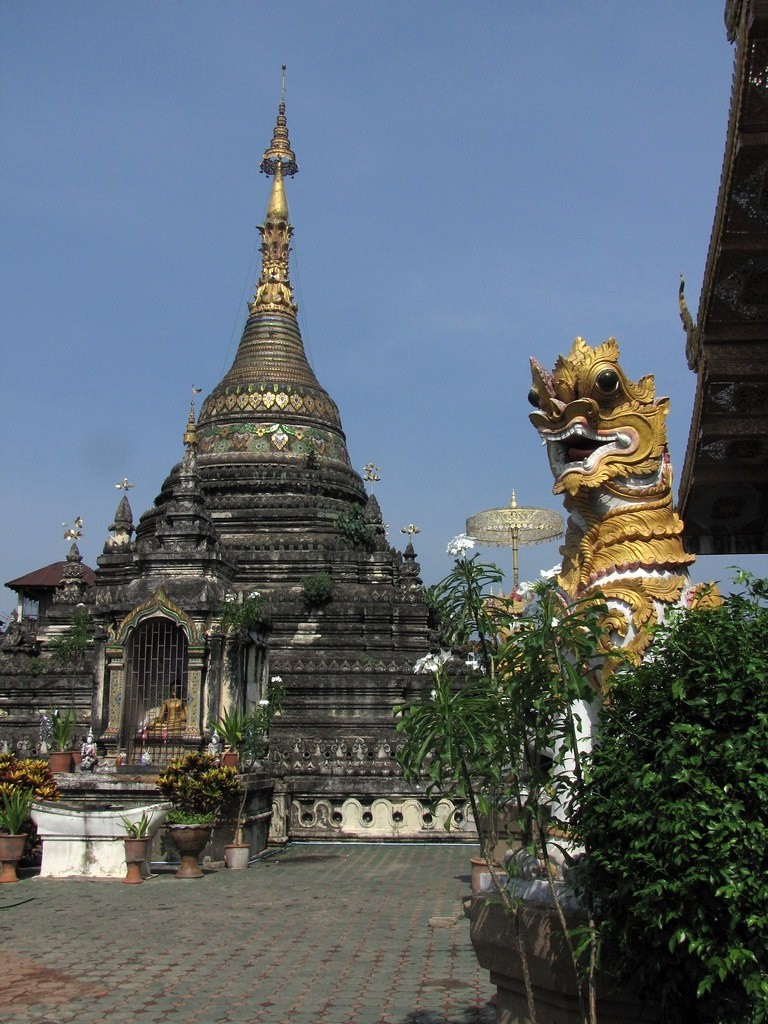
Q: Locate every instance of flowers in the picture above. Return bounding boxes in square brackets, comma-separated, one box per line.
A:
[234, 675, 290, 846]
[0, 750, 65, 804]
[156, 750, 239, 823]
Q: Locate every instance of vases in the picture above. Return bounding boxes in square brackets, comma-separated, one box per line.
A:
[469, 858, 507, 894]
[72, 750, 81, 766]
[224, 843, 252, 870]
[168, 823, 215, 879]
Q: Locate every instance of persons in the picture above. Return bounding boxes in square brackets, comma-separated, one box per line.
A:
[208, 730, 224, 764]
[81, 726, 98, 769]
[146, 682, 187, 735]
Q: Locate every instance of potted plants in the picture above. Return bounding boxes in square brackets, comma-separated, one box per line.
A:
[0, 790, 34, 885]
[47, 705, 77, 772]
[209, 707, 250, 771]
[117, 808, 154, 883]
